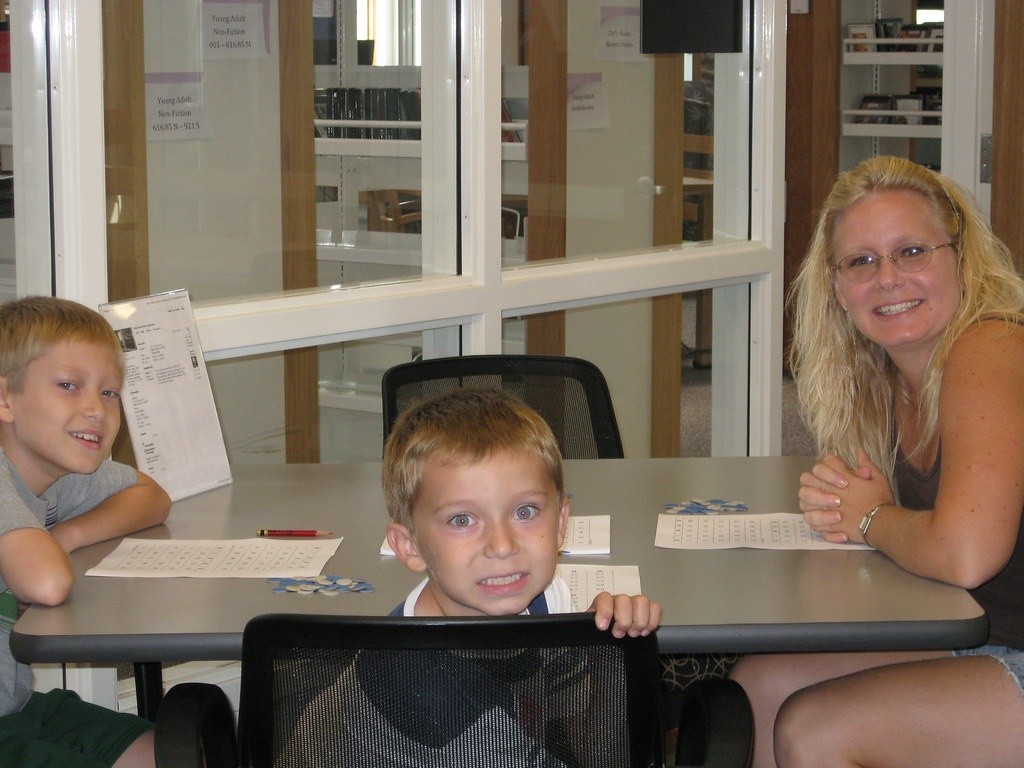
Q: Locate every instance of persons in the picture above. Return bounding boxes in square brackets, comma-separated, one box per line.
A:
[279, 386, 666, 768]
[735, 155, 1024, 768]
[0, 296, 172, 768]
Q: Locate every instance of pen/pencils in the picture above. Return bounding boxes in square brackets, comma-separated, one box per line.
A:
[255, 528, 337, 538]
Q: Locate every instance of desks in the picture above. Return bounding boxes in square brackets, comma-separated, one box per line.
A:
[676, 173, 712, 367]
[7, 455, 989, 717]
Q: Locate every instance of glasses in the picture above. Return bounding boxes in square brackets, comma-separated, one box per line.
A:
[832, 242, 958, 283]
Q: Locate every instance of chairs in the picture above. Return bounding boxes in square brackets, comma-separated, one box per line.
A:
[369, 182, 527, 242]
[381, 354, 627, 461]
[155, 614, 757, 768]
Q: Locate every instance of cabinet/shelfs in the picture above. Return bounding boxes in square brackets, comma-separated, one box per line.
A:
[839, 0, 948, 183]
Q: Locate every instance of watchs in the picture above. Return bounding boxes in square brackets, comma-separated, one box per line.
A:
[859, 503, 895, 548]
